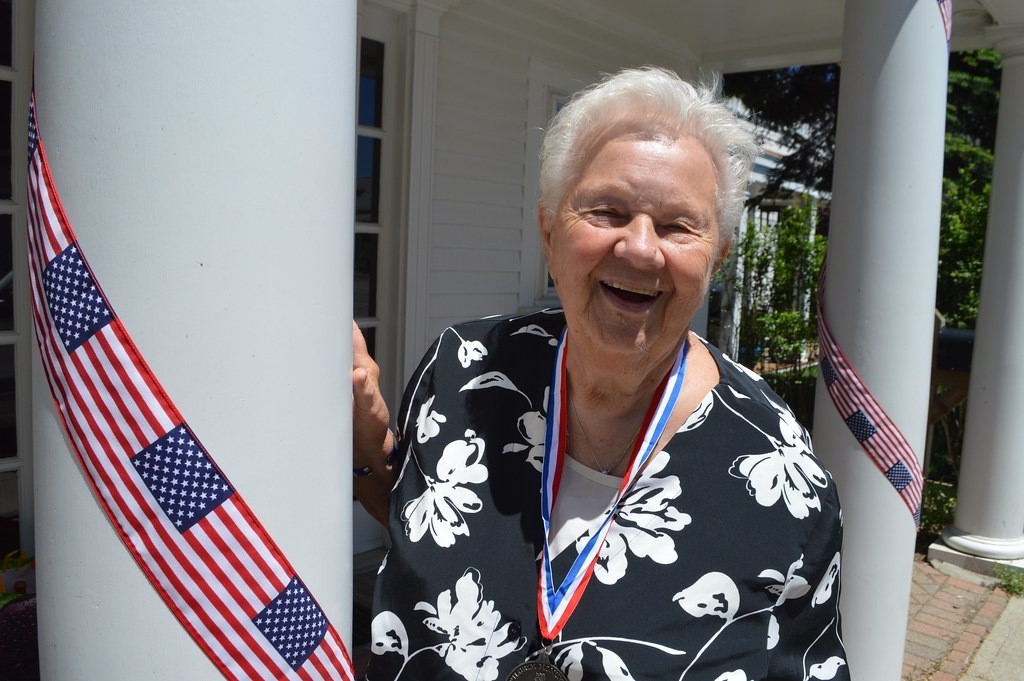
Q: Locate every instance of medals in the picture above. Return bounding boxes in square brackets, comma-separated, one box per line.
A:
[508, 638, 570, 681]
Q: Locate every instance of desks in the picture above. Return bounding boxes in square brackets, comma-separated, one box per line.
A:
[923, 370, 972, 465]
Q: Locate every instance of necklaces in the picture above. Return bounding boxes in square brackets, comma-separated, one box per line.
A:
[565, 368, 646, 475]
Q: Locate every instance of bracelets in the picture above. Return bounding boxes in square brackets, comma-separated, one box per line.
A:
[353, 450, 394, 478]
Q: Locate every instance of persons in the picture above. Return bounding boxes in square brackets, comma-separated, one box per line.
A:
[354, 64, 852, 681]
